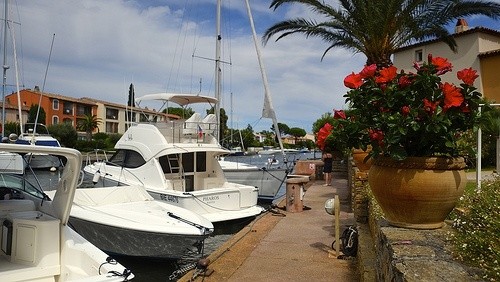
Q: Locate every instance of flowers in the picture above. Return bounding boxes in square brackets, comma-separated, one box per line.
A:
[313, 52, 497, 163]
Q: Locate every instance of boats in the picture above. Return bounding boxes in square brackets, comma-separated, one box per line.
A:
[0, 117, 267, 282]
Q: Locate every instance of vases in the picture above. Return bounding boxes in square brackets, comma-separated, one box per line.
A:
[368, 153, 468, 230]
[351, 145, 374, 170]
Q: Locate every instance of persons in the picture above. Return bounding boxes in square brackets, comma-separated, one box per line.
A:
[273, 157, 276, 163]
[322, 149, 333, 186]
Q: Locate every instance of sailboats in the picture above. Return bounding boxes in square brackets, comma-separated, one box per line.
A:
[226, 93, 245, 156]
[127, 0, 300, 203]
[0, 0, 63, 176]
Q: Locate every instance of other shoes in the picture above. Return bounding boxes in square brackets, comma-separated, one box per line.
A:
[323, 183, 332, 186]
